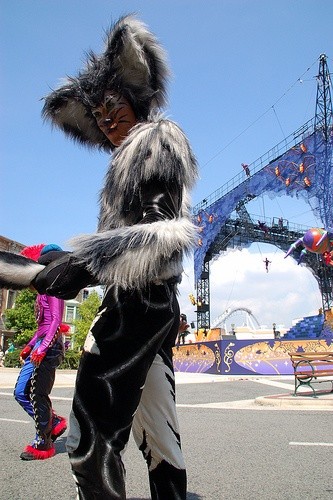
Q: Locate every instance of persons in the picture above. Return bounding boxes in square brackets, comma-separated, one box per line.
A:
[263, 258, 272, 274]
[13, 243, 69, 461]
[241, 163, 251, 178]
[30, 11, 198, 500]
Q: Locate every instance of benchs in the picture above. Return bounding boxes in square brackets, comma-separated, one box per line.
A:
[288, 351, 333, 399]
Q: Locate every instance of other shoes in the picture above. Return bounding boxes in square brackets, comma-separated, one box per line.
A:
[46, 414, 67, 443]
[19, 445, 54, 460]
